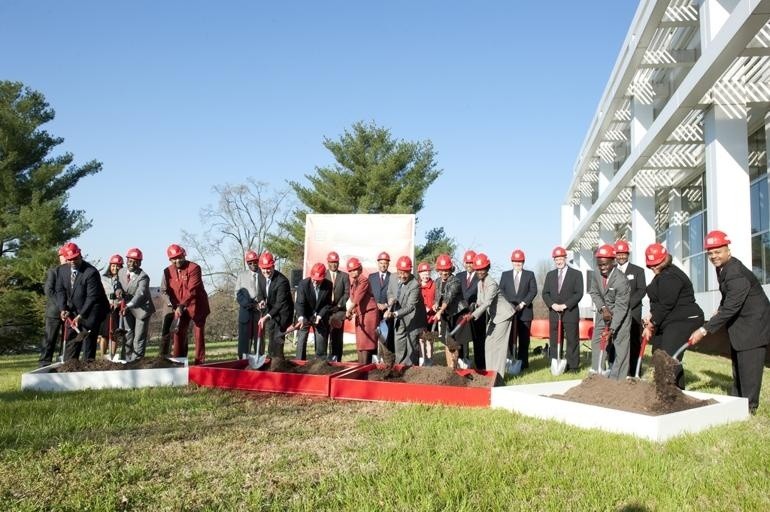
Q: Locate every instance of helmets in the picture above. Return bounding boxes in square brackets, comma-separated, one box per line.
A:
[168, 244, 186, 259]
[58, 243, 80, 259]
[126, 248, 142, 260]
[552, 247, 566, 256]
[464, 250, 490, 269]
[311, 252, 360, 280]
[596, 241, 629, 258]
[246, 251, 274, 268]
[646, 243, 667, 266]
[511, 250, 524, 261]
[109, 255, 124, 264]
[377, 252, 452, 272]
[704, 231, 730, 248]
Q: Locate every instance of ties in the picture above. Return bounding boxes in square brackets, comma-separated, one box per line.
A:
[558, 271, 563, 293]
[71, 272, 76, 288]
[467, 273, 471, 288]
[603, 275, 607, 288]
[380, 274, 384, 285]
[514, 272, 519, 294]
[127, 273, 130, 283]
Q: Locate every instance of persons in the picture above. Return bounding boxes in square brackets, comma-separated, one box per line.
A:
[641, 243, 704, 391]
[498, 250, 538, 370]
[102, 247, 156, 361]
[40, 242, 111, 363]
[158, 244, 211, 363]
[589, 244, 630, 380]
[234, 252, 295, 358]
[293, 251, 516, 378]
[542, 247, 583, 372]
[606, 241, 643, 377]
[689, 230, 770, 416]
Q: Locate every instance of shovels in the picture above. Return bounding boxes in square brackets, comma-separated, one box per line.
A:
[326, 331, 338, 363]
[626, 323, 649, 383]
[168, 308, 181, 334]
[242, 320, 253, 360]
[506, 310, 523, 376]
[378, 298, 394, 343]
[274, 315, 316, 345]
[445, 303, 478, 346]
[247, 304, 267, 370]
[55, 298, 127, 363]
[550, 310, 568, 376]
[331, 311, 351, 328]
[659, 339, 692, 380]
[424, 316, 440, 366]
[588, 317, 611, 379]
